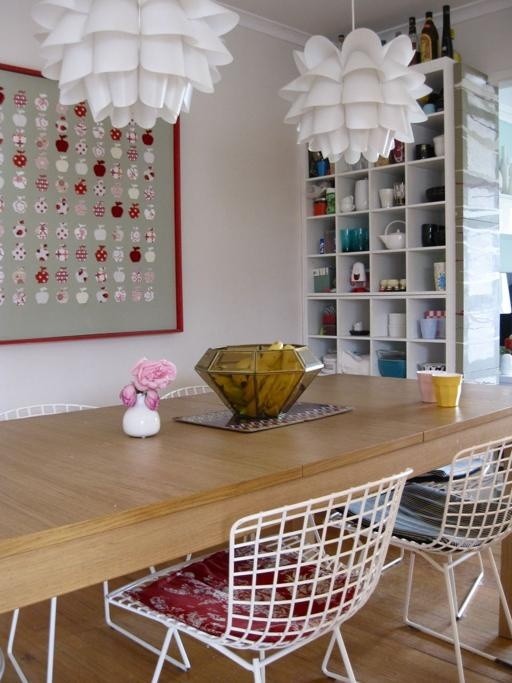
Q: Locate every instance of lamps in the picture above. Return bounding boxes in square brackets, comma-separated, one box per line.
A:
[278, 0, 433, 166]
[27, 0, 240, 130]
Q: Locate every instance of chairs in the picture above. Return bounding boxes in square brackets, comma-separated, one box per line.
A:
[2, 403, 192, 682]
[106, 467, 415, 681]
[404, 376, 498, 620]
[158, 385, 214, 398]
[320, 434, 511, 681]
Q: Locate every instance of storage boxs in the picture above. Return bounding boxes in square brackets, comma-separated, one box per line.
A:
[312, 266, 335, 292]
[425, 310, 446, 341]
[377, 349, 406, 378]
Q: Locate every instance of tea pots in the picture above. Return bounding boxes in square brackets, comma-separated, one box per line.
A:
[378, 220, 405, 249]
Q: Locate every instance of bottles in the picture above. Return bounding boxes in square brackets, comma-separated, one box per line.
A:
[420, 10, 438, 62]
[406, 15, 418, 66]
[441, 4, 453, 57]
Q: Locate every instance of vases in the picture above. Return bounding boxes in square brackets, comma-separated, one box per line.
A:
[432, 134, 445, 158]
[388, 313, 406, 340]
[414, 144, 434, 161]
[419, 319, 438, 341]
[433, 263, 446, 291]
[123, 393, 162, 436]
[379, 188, 394, 210]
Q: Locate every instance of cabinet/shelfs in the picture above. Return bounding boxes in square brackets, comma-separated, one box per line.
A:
[298, 56, 500, 387]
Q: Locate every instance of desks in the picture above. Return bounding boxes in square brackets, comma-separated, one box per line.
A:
[2, 374, 511, 604]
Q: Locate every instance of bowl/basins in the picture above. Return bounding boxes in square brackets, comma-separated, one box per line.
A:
[425, 186, 445, 203]
[432, 135, 446, 157]
[421, 223, 443, 246]
[415, 143, 432, 159]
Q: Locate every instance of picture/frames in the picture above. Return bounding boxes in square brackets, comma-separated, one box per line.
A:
[1, 63, 184, 345]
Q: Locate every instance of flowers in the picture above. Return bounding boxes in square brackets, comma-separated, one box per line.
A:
[120, 355, 176, 408]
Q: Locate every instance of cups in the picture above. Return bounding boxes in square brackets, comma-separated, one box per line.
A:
[418, 319, 437, 339]
[415, 369, 443, 403]
[349, 228, 358, 250]
[431, 370, 463, 407]
[379, 188, 394, 210]
[342, 195, 355, 211]
[356, 227, 368, 250]
[341, 227, 353, 251]
[433, 261, 445, 291]
